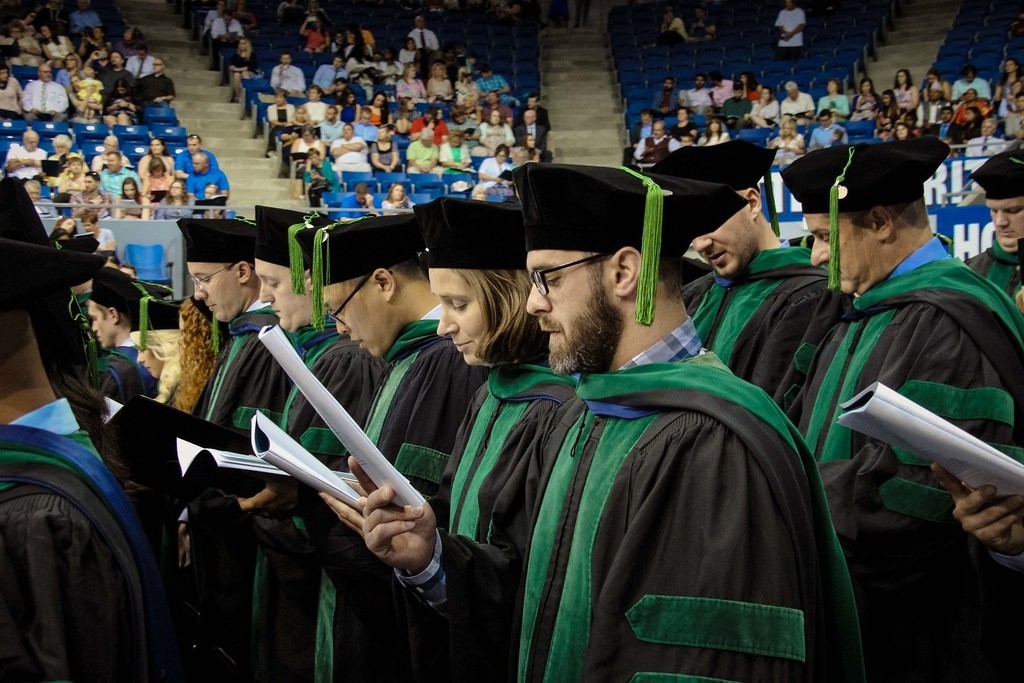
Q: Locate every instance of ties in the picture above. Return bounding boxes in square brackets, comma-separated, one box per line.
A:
[41, 83, 47, 113]
[420, 31, 425, 48]
[982, 138, 988, 152]
[136, 60, 144, 78]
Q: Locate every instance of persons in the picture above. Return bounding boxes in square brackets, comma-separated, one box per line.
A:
[653, 0, 716, 46]
[202, 0, 592, 221]
[0, 0, 230, 280]
[623, 58, 1024, 158]
[774, 0, 806, 59]
[0, 139, 1024, 683]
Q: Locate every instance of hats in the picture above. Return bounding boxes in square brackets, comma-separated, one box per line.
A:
[92, 266, 172, 319]
[0, 176, 52, 249]
[127, 296, 179, 350]
[512, 161, 750, 326]
[53, 236, 101, 253]
[968, 149, 1024, 200]
[651, 139, 780, 237]
[1, 237, 108, 392]
[190, 294, 231, 358]
[254, 205, 338, 296]
[450, 105, 466, 117]
[779, 137, 951, 292]
[412, 196, 528, 270]
[293, 214, 419, 333]
[176, 214, 258, 264]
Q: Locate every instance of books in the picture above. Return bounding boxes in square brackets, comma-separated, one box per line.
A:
[192, 195, 228, 214]
[175, 437, 360, 499]
[258, 324, 427, 508]
[74, 231, 94, 239]
[783, 111, 810, 117]
[41, 153, 67, 177]
[836, 381, 1024, 500]
[102, 394, 252, 464]
[498, 167, 516, 181]
[151, 189, 168, 203]
[119, 199, 141, 214]
[250, 409, 364, 511]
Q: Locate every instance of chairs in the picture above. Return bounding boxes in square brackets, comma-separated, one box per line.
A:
[169, 0, 552, 220]
[600, 0, 1024, 175]
[0, 0, 234, 285]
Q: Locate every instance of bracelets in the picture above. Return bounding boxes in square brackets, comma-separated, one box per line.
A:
[128, 103, 129, 106]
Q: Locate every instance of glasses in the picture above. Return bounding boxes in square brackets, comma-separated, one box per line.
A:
[190, 260, 241, 291]
[528, 253, 609, 295]
[39, 71, 53, 74]
[328, 269, 395, 327]
